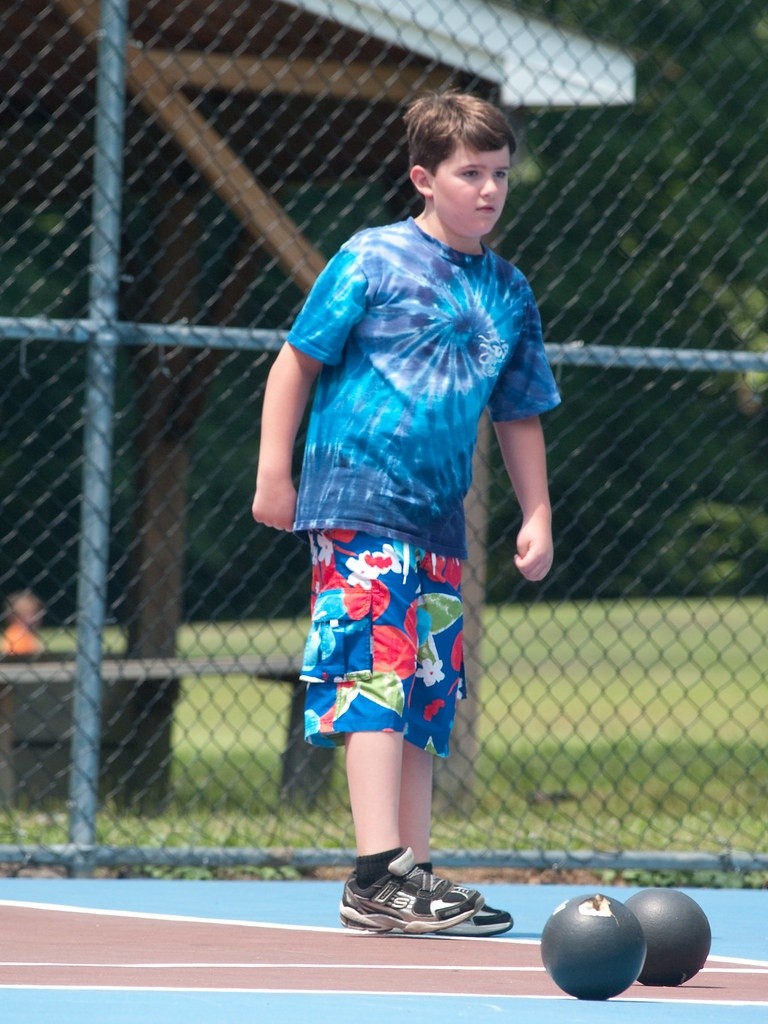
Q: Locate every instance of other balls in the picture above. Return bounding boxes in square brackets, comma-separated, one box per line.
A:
[621, 887, 714, 988]
[539, 893, 648, 1001]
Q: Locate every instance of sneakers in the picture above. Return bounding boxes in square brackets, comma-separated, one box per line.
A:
[438, 903, 513, 935]
[339, 846, 485, 935]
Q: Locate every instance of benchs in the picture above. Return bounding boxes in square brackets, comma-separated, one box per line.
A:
[0, 652, 335, 807]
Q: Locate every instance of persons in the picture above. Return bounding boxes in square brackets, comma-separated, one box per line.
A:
[250, 93, 562, 935]
[0, 595, 43, 652]
